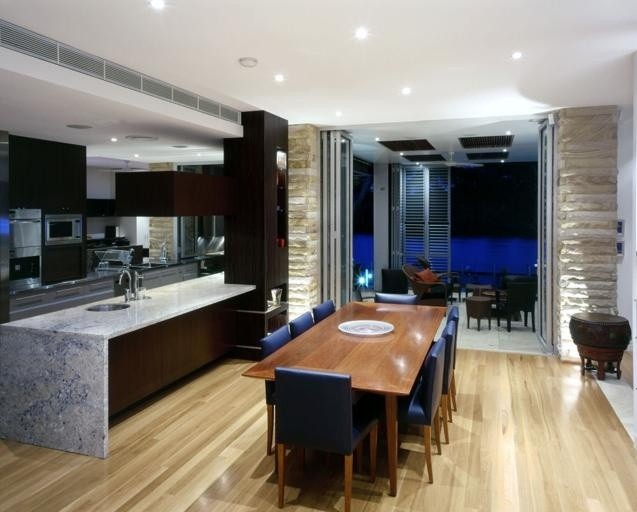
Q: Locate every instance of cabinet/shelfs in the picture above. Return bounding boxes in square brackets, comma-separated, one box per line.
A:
[86, 277, 116, 308]
[162, 263, 200, 289]
[6, 290, 48, 324]
[141, 268, 163, 293]
[224, 110, 290, 363]
[48, 283, 86, 314]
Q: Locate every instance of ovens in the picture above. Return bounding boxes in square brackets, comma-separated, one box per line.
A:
[9, 208, 42, 295]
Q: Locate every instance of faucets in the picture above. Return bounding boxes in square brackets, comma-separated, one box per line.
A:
[118, 270, 132, 298]
[133, 270, 143, 299]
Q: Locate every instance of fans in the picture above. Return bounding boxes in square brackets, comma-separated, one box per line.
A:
[401, 151, 482, 168]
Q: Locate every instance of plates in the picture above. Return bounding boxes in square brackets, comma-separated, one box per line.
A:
[337, 319, 394, 337]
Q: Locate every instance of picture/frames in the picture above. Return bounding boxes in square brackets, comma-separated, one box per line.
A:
[177, 165, 197, 260]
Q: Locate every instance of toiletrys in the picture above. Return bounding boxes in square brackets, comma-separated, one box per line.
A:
[160, 240, 168, 261]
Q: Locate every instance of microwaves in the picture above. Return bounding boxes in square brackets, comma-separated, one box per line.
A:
[45, 219, 82, 240]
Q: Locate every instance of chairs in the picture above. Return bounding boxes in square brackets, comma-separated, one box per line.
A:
[402, 263, 453, 305]
[495, 281, 537, 332]
[272, 367, 378, 511]
[258, 293, 460, 484]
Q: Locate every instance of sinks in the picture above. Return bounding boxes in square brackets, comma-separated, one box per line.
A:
[86, 303, 130, 312]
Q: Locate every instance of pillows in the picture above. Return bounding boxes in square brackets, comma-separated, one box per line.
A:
[416, 268, 438, 291]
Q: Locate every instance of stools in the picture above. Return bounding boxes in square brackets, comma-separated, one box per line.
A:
[466, 296, 493, 332]
[570, 313, 632, 382]
